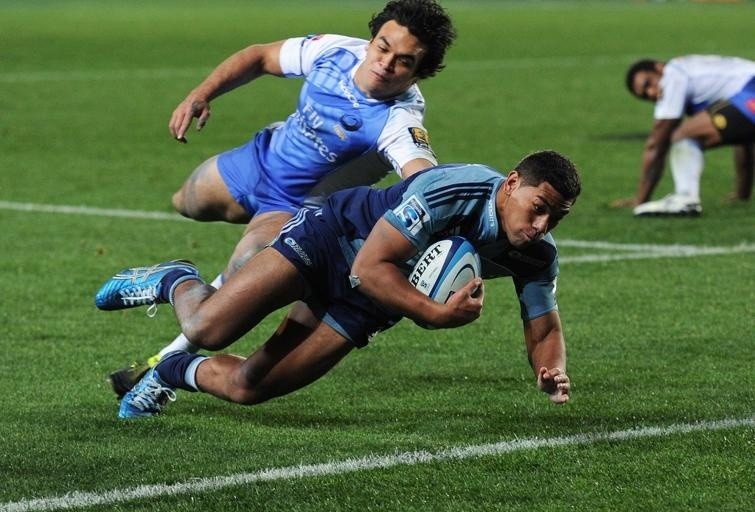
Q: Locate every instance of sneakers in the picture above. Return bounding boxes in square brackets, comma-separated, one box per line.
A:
[91, 256, 206, 317]
[106, 353, 162, 400]
[631, 193, 703, 217]
[117, 346, 190, 420]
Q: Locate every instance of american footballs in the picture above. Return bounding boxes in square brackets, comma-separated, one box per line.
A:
[406, 236, 481, 330]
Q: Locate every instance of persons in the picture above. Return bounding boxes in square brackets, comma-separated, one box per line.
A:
[625, 55, 755, 214]
[107, 1, 458, 397]
[93, 148, 582, 419]
